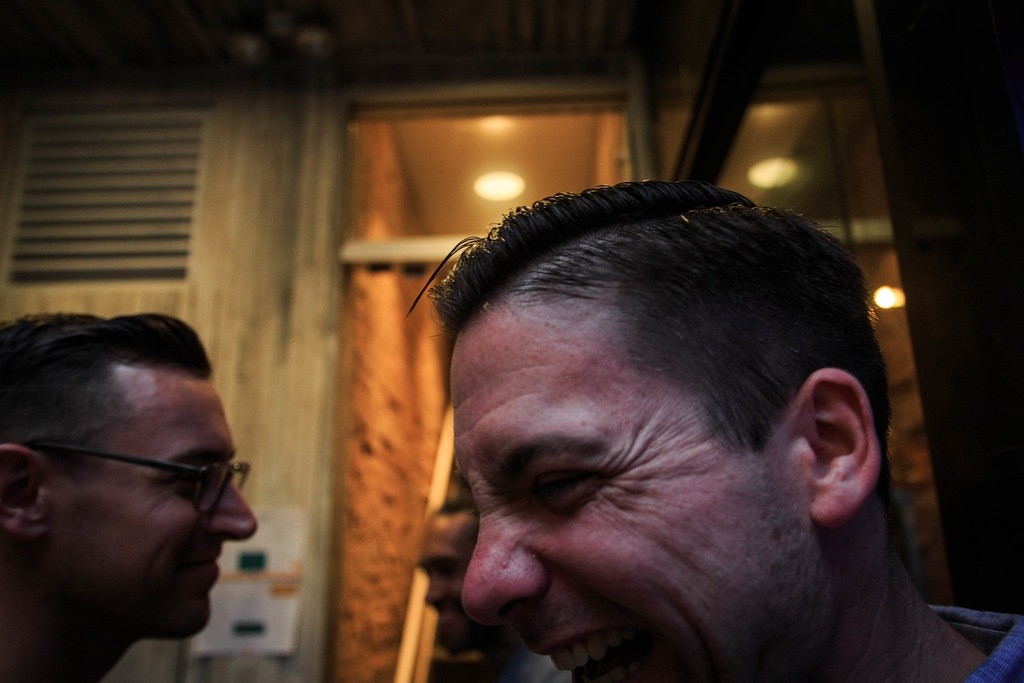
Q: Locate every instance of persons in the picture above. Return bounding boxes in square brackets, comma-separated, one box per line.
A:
[0, 311, 259, 683]
[404, 180, 1024, 683]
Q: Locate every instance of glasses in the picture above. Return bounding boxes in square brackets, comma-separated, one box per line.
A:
[26, 437, 249, 515]
